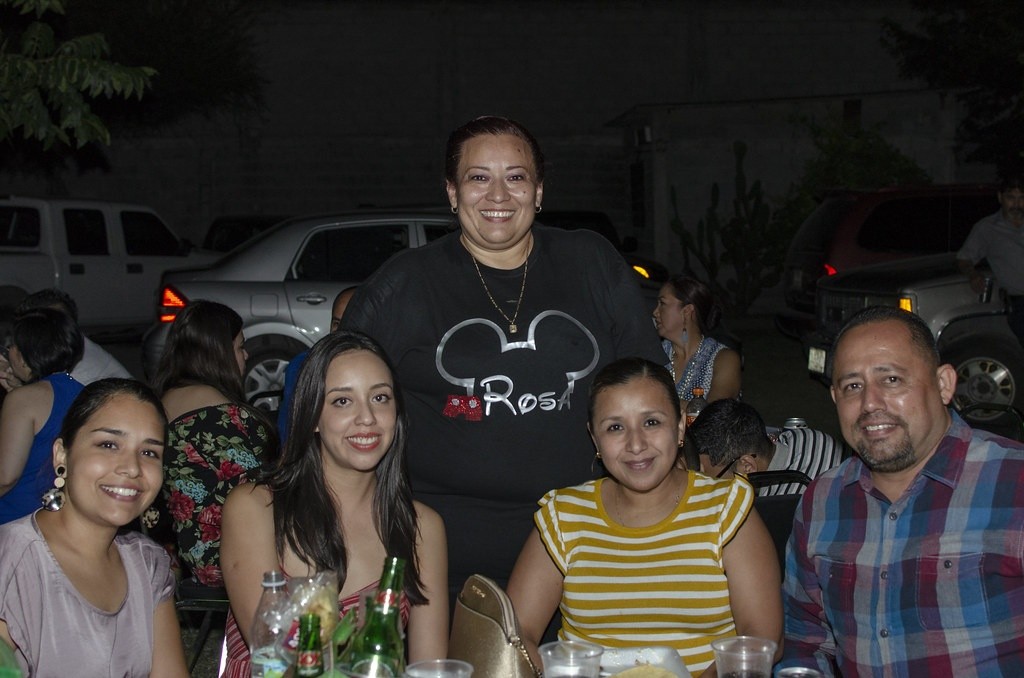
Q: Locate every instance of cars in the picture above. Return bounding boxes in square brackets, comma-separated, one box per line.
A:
[142, 209, 747, 422]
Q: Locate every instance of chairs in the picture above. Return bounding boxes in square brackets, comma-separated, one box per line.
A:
[747, 470, 812, 583]
[139, 512, 229, 674]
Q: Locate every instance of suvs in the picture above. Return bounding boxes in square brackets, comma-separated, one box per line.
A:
[773, 178, 1024, 433]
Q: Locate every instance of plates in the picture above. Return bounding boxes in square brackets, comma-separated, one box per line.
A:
[602, 646, 693, 678]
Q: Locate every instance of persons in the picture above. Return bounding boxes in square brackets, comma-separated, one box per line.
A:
[0, 287, 134, 526]
[0, 377, 190, 678]
[507, 358, 786, 678]
[954, 175, 1024, 346]
[687, 401, 847, 496]
[278, 286, 358, 448]
[219, 331, 449, 678]
[653, 278, 742, 423]
[149, 301, 282, 592]
[783, 305, 1024, 677]
[341, 115, 666, 643]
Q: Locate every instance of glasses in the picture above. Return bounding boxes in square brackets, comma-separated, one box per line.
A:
[717, 453, 756, 479]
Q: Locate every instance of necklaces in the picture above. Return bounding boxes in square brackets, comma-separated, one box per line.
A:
[671, 334, 704, 398]
[614, 490, 680, 526]
[473, 248, 528, 333]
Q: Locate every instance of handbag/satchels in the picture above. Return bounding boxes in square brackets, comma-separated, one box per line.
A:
[448, 572, 542, 678]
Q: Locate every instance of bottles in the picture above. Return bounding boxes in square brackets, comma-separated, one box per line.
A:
[0, 636, 24, 678]
[346, 557, 410, 678]
[248, 571, 291, 677]
[686, 387, 708, 429]
[293, 615, 324, 678]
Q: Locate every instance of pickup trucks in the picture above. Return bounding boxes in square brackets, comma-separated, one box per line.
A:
[0, 193, 228, 332]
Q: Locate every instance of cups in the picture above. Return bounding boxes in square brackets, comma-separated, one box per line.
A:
[358, 591, 405, 643]
[405, 658, 473, 678]
[711, 636, 778, 678]
[538, 640, 604, 678]
